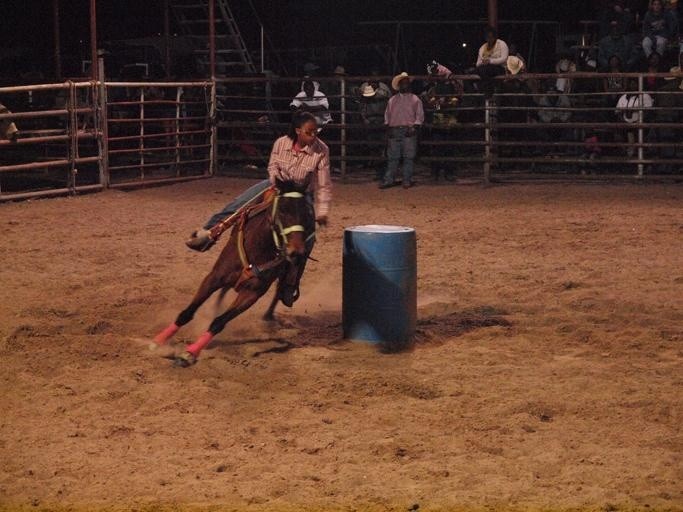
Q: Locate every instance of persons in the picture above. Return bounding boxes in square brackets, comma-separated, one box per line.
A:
[186, 110, 332, 307]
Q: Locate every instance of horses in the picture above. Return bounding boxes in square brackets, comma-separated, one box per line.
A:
[1, 103, 20, 142]
[146, 167, 315, 367]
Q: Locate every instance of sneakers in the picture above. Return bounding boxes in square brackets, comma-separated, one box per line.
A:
[378, 181, 413, 190]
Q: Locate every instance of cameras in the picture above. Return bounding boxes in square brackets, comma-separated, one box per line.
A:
[424, 63, 439, 72]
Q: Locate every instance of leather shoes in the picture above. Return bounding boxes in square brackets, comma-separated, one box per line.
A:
[182, 234, 213, 252]
[280, 284, 294, 308]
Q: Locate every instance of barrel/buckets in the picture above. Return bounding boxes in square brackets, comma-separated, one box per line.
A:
[342, 225, 417, 343]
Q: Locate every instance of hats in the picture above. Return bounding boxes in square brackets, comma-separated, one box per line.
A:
[360, 85, 376, 99]
[326, 65, 348, 77]
[663, 66, 683, 82]
[506, 55, 523, 75]
[390, 71, 415, 92]
